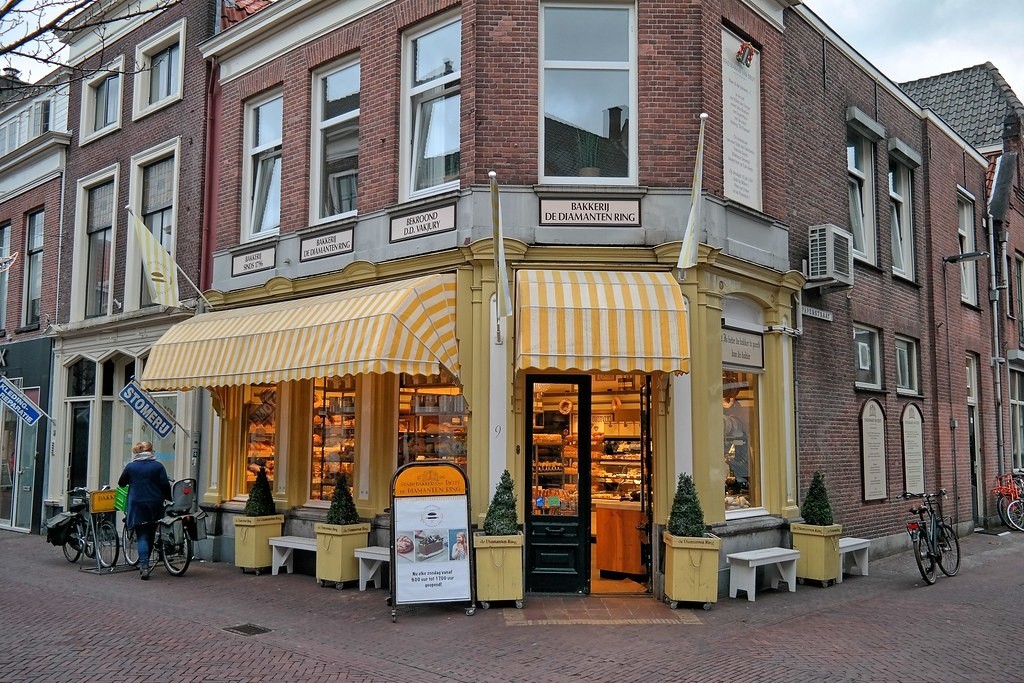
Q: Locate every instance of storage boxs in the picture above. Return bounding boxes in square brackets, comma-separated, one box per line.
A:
[114, 484, 129, 512]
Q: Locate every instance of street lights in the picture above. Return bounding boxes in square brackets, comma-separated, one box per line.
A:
[941, 251, 991, 540]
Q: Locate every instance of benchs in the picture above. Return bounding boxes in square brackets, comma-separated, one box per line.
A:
[354, 546, 391, 591]
[267, 536, 318, 576]
[727, 547, 800, 602]
[838, 536, 873, 584]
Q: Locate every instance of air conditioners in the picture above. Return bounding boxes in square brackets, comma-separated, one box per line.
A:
[801, 223, 854, 294]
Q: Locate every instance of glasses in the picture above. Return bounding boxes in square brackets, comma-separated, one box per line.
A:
[150, 450, 155, 453]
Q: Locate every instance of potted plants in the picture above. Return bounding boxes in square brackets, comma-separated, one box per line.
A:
[790, 471, 842, 588]
[233, 464, 283, 574]
[473, 469, 527, 609]
[314, 472, 371, 589]
[662, 473, 722, 611]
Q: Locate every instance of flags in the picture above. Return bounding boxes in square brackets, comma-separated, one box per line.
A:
[497, 193, 512, 322]
[133, 215, 179, 308]
[676, 130, 703, 268]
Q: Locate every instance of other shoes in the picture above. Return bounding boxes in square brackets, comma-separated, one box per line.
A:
[139, 566, 149, 579]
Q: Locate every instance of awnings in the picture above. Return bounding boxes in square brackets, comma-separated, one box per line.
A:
[140, 273, 464, 409]
[515, 270, 689, 376]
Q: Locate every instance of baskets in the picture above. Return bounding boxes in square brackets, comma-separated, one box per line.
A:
[114, 485, 130, 513]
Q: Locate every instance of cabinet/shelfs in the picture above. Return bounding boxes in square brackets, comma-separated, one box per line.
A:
[245, 394, 648, 516]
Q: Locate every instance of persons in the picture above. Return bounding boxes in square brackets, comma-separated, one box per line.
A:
[119, 441, 172, 581]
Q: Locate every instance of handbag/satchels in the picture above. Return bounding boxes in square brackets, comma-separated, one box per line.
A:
[45, 510, 95, 546]
[157, 509, 209, 545]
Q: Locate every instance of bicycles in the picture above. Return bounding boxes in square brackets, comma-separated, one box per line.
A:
[122, 476, 195, 577]
[896, 488, 961, 585]
[992, 467, 1024, 532]
[60, 485, 119, 573]
